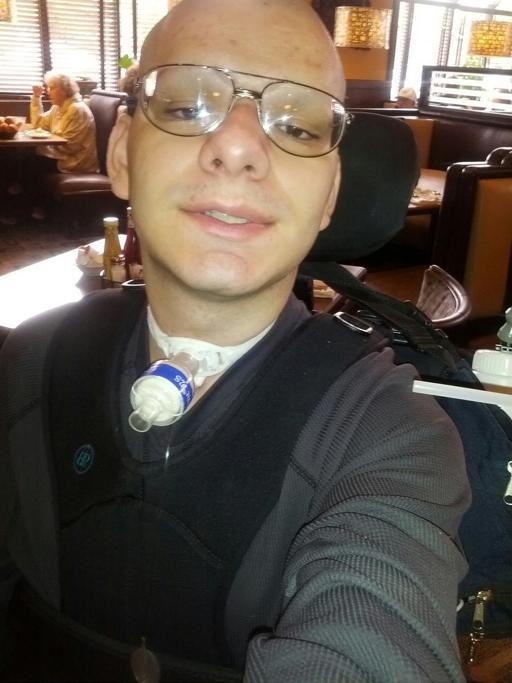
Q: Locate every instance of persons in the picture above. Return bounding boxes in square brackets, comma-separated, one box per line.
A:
[0, 0, 474, 683]
[30, 68, 101, 175]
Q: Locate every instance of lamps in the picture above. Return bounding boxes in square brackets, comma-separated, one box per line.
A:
[467, 20, 512, 56]
[332, 6, 394, 51]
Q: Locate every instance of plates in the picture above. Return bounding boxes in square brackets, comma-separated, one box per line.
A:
[26, 129, 51, 140]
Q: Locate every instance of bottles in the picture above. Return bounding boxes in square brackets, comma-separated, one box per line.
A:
[101, 205, 143, 290]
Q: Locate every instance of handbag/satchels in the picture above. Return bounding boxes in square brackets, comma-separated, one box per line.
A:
[341, 289, 512, 640]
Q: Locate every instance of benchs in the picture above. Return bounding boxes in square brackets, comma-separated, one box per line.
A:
[44, 89, 127, 237]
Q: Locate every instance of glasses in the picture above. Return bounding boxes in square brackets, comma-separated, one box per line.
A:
[126, 64, 355, 158]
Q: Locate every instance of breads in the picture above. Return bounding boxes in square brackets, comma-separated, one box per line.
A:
[0, 117, 18, 133]
